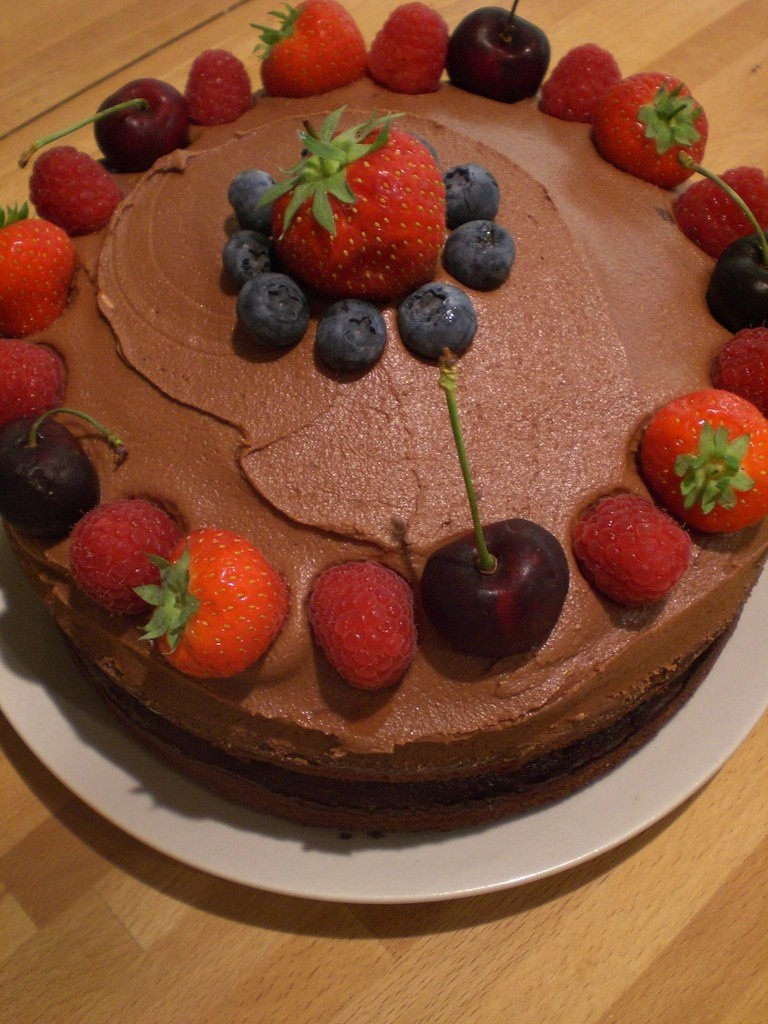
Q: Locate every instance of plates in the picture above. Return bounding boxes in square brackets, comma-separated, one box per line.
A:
[0, 522, 768, 904]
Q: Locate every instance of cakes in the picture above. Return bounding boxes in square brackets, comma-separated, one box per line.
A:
[0, 0, 768, 837]
